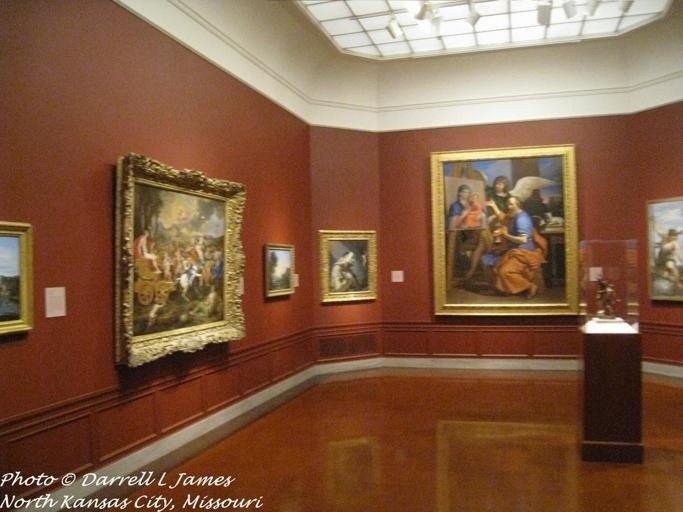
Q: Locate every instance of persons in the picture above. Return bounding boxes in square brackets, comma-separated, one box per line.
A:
[650, 225, 682, 291]
[444, 172, 565, 299]
[593, 277, 619, 318]
[327, 250, 359, 291]
[131, 222, 224, 322]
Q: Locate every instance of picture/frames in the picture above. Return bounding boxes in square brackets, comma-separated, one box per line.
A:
[429, 143, 579, 317]
[0, 221, 35, 336]
[643, 195, 683, 303]
[316, 229, 378, 307]
[114, 152, 248, 369]
[264, 242, 295, 298]
